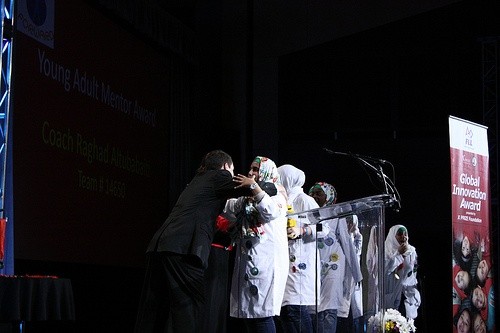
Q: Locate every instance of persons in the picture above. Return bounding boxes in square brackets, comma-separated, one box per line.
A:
[454, 229, 497, 333]
[276, 162, 323, 332]
[339, 211, 364, 333]
[381, 226, 422, 332]
[304, 180, 369, 333]
[143, 150, 288, 333]
[215, 152, 288, 333]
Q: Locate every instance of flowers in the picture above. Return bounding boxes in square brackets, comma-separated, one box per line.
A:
[368, 307, 417, 333]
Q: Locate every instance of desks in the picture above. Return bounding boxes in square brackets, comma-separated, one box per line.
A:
[0, 274, 78, 333]
